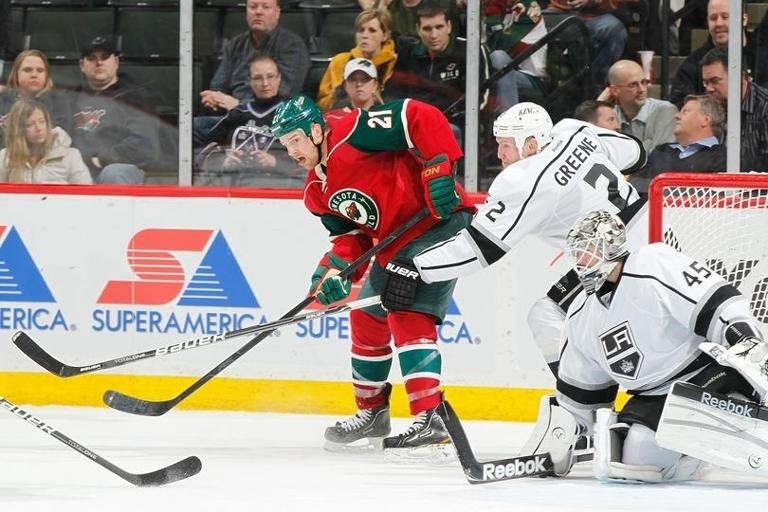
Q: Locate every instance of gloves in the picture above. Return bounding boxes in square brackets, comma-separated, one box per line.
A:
[380, 257, 421, 312]
[309, 251, 352, 305]
[420, 154, 462, 221]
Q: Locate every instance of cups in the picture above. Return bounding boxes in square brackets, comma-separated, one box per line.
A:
[637, 50, 655, 84]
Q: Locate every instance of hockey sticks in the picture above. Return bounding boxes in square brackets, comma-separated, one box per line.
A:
[103, 208, 430, 417]
[12, 296, 382, 377]
[0, 397, 201, 486]
[441, 13, 623, 174]
[435, 401, 594, 486]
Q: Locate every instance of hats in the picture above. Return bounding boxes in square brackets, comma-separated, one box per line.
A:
[344, 57, 378, 81]
[81, 34, 116, 56]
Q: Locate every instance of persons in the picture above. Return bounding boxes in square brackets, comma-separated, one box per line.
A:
[526, 204, 767, 483]
[191, 2, 767, 194]
[62, 34, 161, 186]
[0, 49, 70, 145]
[0, 95, 91, 184]
[268, 93, 474, 451]
[380, 99, 654, 450]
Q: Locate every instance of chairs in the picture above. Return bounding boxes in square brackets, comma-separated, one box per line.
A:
[0, 2, 578, 186]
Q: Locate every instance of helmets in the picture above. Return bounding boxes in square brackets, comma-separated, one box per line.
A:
[270, 95, 326, 140]
[565, 210, 630, 296]
[493, 102, 554, 147]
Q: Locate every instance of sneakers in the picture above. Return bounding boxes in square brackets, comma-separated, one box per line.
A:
[324, 403, 391, 444]
[383, 409, 453, 449]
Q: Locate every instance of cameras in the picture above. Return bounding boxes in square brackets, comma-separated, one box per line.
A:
[238, 156, 255, 168]
